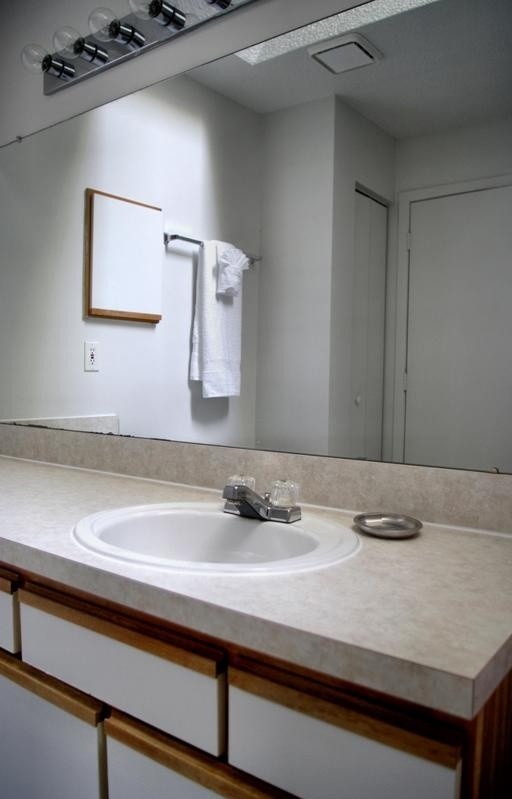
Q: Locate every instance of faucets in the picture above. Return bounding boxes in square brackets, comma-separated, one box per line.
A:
[222, 484, 272, 522]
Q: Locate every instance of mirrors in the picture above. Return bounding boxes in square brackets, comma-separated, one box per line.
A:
[0, 0, 512, 476]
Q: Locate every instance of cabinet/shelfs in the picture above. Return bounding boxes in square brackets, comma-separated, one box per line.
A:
[0, 559, 512, 799]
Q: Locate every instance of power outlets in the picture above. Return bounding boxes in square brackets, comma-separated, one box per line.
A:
[85, 340, 101, 372]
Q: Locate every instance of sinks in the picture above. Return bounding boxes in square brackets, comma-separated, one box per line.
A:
[72, 502, 363, 578]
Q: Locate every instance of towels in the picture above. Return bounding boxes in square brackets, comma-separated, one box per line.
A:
[189, 236, 251, 400]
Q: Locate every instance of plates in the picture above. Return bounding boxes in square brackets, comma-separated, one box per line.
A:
[352, 510, 424, 539]
